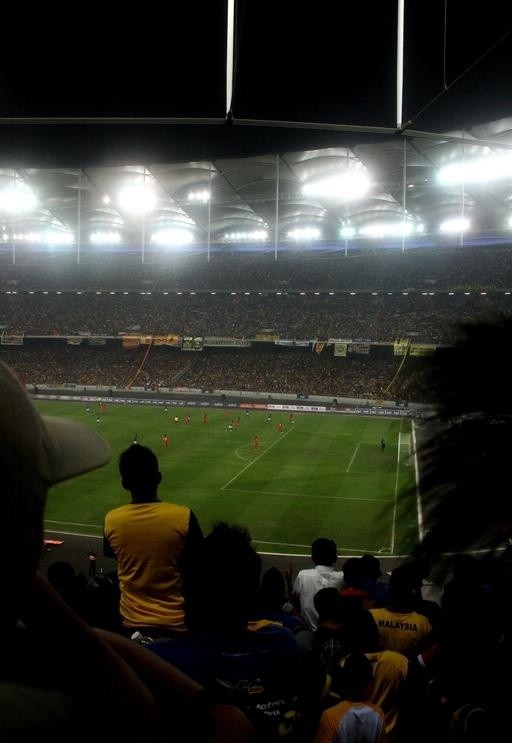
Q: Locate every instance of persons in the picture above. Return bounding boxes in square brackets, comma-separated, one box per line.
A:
[100, 442, 215, 660]
[1, 358, 255, 741]
[1, 516, 512, 742]
[277, 420, 283, 432]
[134, 432, 138, 443]
[253, 434, 259, 450]
[381, 437, 385, 449]
[96, 416, 101, 426]
[0, 244, 512, 423]
[163, 434, 169, 446]
[228, 421, 234, 432]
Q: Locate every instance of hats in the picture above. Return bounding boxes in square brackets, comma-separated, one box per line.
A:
[0, 358, 113, 498]
